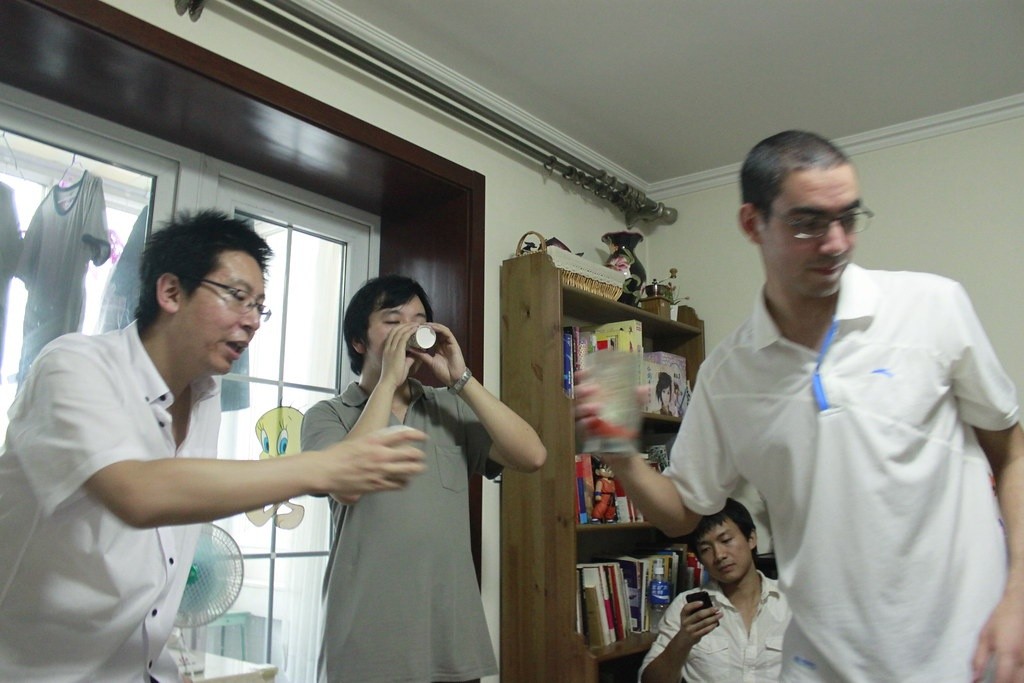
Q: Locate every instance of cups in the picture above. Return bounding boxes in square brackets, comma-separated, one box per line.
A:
[385, 423, 421, 485]
[406, 324, 437, 350]
[582, 349, 642, 455]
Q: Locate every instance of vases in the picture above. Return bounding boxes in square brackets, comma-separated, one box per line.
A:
[602, 231, 647, 307]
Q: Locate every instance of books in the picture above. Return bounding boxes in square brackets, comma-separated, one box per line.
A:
[563, 320, 712, 648]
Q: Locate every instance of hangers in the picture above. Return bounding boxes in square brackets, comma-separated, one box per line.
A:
[0, 129, 25, 193]
[57, 153, 86, 190]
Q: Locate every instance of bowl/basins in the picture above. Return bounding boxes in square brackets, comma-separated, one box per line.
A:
[644, 278, 670, 297]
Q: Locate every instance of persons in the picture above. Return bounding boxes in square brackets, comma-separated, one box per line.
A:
[298, 273, 548, 683]
[0, 209, 432, 683]
[590, 462, 620, 524]
[570, 127, 1024, 683]
[637, 497, 793, 683]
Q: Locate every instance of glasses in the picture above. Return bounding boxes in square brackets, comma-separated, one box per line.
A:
[175, 272, 271, 323]
[764, 206, 873, 239]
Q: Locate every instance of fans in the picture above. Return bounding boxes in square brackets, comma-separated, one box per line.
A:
[164, 524, 245, 674]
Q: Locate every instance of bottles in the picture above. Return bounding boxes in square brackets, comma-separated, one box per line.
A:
[646, 568, 670, 634]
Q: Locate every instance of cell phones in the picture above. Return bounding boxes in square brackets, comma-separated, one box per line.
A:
[686, 592, 720, 627]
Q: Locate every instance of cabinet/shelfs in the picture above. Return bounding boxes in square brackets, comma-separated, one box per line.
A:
[498, 253, 706, 683]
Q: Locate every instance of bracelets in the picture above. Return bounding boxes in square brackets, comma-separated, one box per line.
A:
[447, 367, 473, 395]
